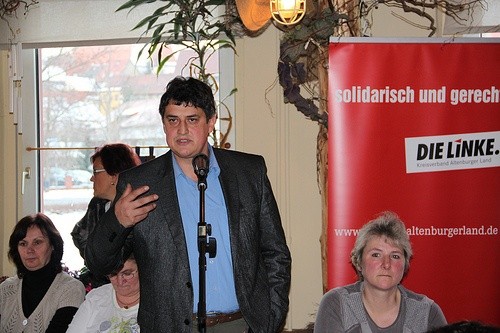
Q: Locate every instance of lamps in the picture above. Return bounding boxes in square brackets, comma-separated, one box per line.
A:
[269, 0, 306, 26]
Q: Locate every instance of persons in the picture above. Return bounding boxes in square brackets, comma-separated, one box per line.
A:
[0, 142, 144, 333]
[85, 75, 292, 333]
[313, 211, 448, 333]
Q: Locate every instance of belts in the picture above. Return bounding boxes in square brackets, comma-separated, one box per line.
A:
[194, 311, 243, 326]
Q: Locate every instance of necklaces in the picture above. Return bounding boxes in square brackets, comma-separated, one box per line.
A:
[116, 296, 140, 310]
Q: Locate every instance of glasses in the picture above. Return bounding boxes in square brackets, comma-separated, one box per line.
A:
[92, 168, 108, 175]
[106, 268, 138, 280]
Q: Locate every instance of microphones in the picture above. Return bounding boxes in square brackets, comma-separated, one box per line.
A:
[192, 153, 209, 190]
